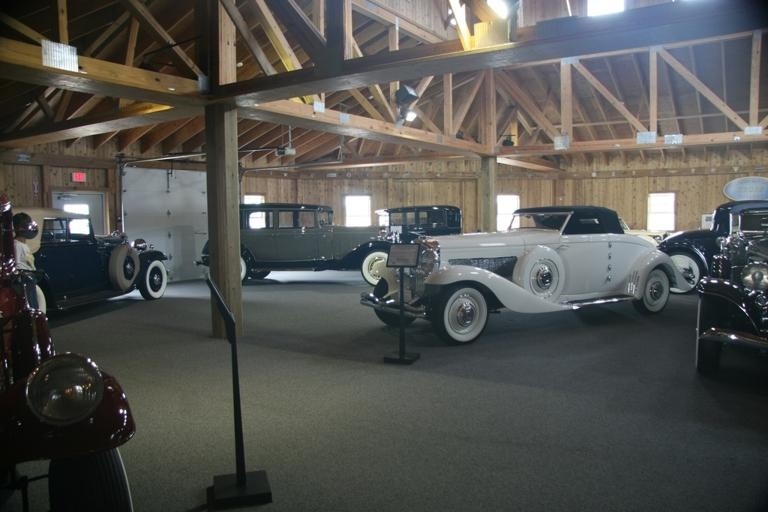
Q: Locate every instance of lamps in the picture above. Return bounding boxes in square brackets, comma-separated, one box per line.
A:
[395, 84, 419, 122]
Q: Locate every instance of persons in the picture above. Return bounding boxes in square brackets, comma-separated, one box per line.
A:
[14, 213, 48, 314]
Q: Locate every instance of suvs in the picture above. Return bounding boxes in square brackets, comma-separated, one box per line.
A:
[193, 203, 389, 286]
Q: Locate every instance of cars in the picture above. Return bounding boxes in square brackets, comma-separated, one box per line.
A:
[657, 200, 766, 379]
[360, 204, 695, 346]
[378, 204, 462, 236]
